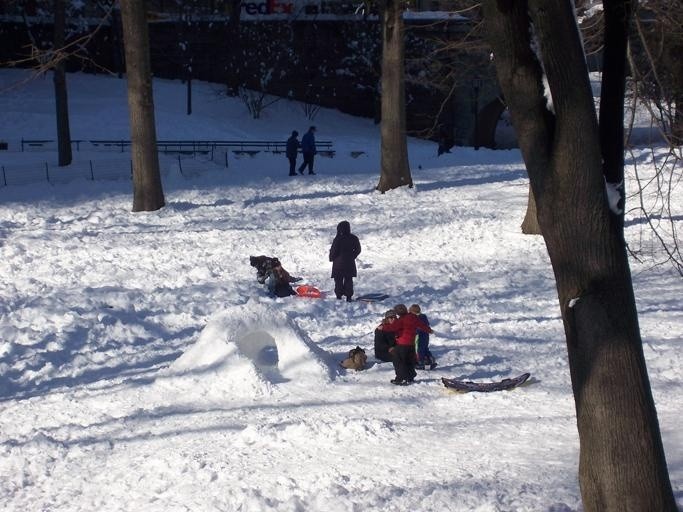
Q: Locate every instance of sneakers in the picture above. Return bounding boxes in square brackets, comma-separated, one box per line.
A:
[391, 373, 417, 384]
[295, 277, 303, 282]
[416, 363, 438, 370]
[289, 168, 316, 176]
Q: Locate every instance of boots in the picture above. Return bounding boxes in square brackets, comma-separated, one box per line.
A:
[337, 295, 356, 302]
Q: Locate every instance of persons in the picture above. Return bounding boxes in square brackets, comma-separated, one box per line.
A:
[265, 261, 304, 298]
[407, 303, 437, 370]
[373, 309, 431, 365]
[298, 125, 317, 175]
[329, 221, 361, 302]
[375, 303, 434, 385]
[285, 131, 302, 177]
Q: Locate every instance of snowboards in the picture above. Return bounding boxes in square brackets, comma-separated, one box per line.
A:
[441, 372, 531, 394]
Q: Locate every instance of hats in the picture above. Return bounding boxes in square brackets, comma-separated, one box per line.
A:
[292, 131, 298, 136]
[385, 310, 396, 318]
[250, 257, 259, 266]
[271, 258, 281, 268]
[395, 304, 407, 314]
[410, 305, 420, 312]
[310, 126, 316, 131]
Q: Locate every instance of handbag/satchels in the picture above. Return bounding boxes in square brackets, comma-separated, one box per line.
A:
[340, 346, 367, 371]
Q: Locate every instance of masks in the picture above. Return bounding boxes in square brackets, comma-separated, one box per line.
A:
[396, 314, 400, 319]
